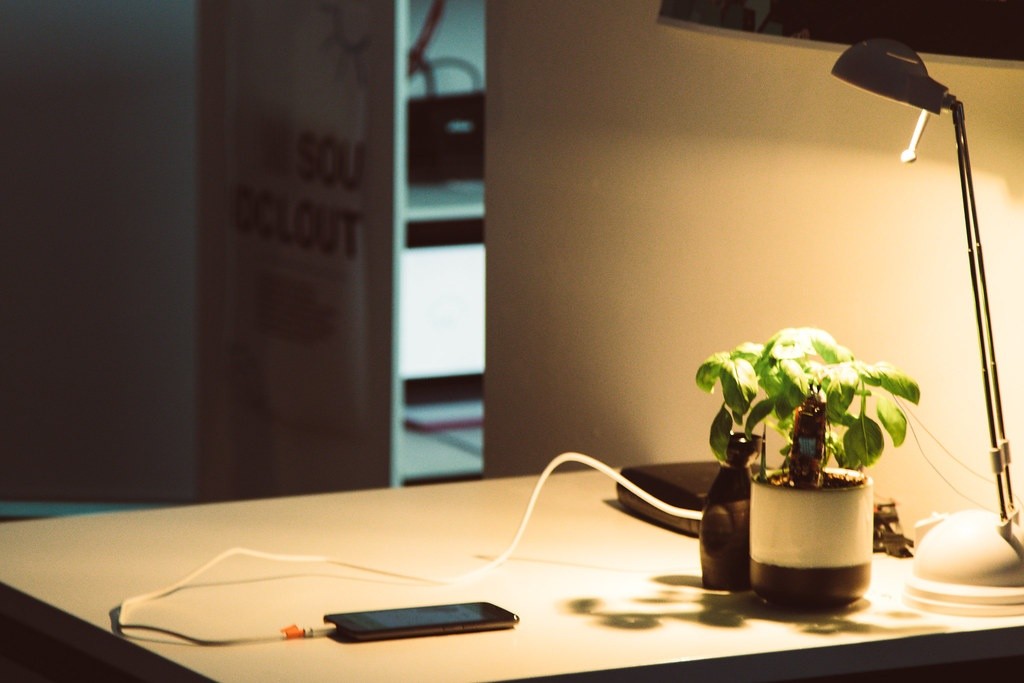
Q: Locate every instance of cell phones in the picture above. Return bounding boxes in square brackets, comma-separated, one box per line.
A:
[323, 602, 519, 641]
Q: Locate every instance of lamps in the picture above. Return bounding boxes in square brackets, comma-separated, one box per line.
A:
[830, 38, 1024, 618]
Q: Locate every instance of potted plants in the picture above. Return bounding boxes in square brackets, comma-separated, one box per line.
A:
[698, 329, 919, 615]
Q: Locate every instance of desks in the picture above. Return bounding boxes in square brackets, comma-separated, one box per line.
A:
[0, 466, 1024, 683]
